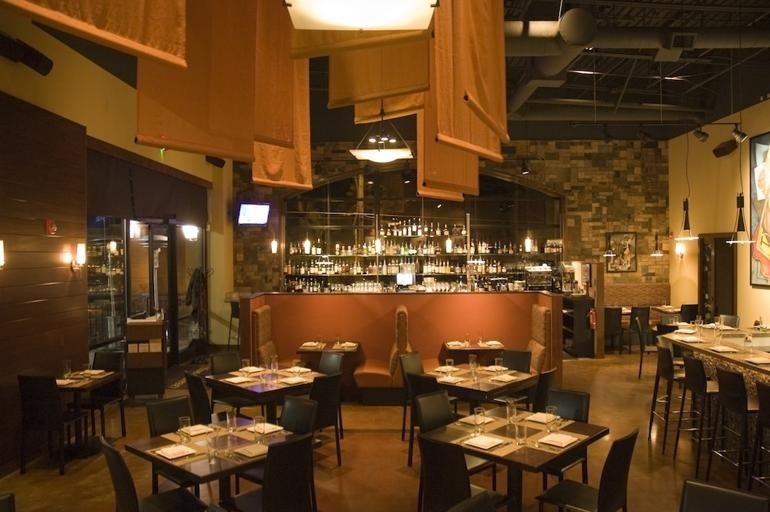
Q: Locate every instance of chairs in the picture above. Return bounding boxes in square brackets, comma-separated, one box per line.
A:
[680, 479, 769, 512]
[0, 493, 15, 511]
[534, 427, 639, 512]
[604, 303, 769, 497]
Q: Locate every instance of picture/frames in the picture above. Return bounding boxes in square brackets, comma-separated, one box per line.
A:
[749, 130, 770, 288]
[606, 232, 638, 272]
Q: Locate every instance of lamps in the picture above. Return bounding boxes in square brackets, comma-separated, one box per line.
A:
[674, 196, 699, 241]
[731, 124, 748, 144]
[349, 99, 414, 164]
[693, 125, 710, 142]
[650, 232, 663, 257]
[72, 243, 86, 272]
[602, 234, 616, 256]
[521, 160, 531, 174]
[726, 192, 756, 243]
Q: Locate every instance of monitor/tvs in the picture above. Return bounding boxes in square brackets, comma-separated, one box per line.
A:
[237, 201, 271, 229]
[396, 273, 414, 290]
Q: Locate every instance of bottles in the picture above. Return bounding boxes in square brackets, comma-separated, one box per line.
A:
[284, 276, 329, 293]
[284, 238, 377, 275]
[378, 217, 523, 275]
[476, 276, 493, 292]
[86, 240, 124, 297]
[544, 239, 562, 254]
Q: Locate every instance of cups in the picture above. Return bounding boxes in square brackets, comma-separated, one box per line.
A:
[241, 359, 301, 381]
[63, 360, 72, 379]
[474, 404, 558, 446]
[661, 299, 666, 308]
[607, 302, 638, 308]
[508, 281, 525, 291]
[179, 407, 265, 465]
[82, 363, 92, 381]
[673, 314, 753, 354]
[446, 354, 503, 384]
[425, 282, 450, 291]
[330, 281, 382, 293]
